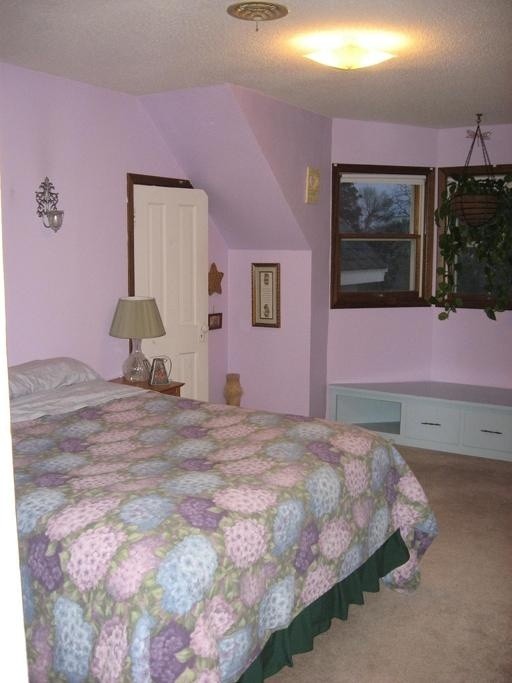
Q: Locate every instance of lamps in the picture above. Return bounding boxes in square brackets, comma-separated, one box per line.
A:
[301, 42, 396, 72]
[109, 293, 167, 384]
[33, 176, 65, 235]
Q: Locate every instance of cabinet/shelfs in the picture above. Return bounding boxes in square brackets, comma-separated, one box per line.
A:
[329, 380, 512, 463]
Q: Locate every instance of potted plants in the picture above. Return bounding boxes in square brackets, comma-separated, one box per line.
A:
[428, 113, 512, 321]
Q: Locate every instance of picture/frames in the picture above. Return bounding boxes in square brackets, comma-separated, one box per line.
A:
[208, 312, 223, 330]
[251, 262, 280, 329]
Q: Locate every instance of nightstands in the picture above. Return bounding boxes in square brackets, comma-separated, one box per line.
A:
[107, 376, 187, 396]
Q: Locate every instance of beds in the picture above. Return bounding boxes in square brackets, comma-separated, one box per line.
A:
[10, 359, 443, 681]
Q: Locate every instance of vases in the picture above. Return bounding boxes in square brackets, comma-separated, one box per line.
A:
[224, 370, 243, 406]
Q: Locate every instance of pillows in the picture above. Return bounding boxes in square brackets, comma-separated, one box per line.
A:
[9, 358, 101, 397]
